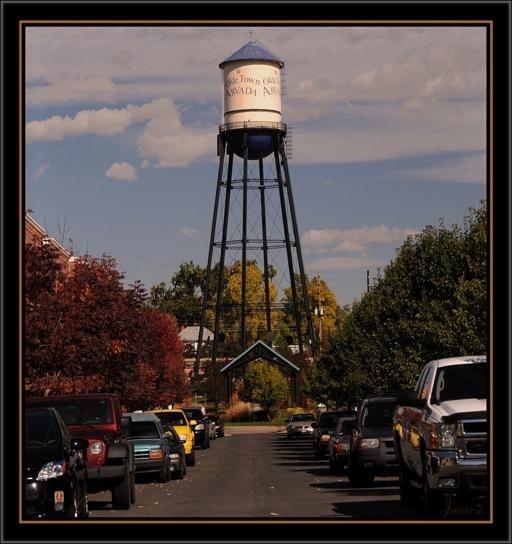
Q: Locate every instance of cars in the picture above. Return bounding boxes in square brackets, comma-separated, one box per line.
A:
[123, 404, 228, 484]
[23, 406, 93, 520]
[283, 410, 359, 469]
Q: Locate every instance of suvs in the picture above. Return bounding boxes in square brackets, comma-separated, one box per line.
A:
[28, 394, 140, 511]
[351, 353, 491, 520]
